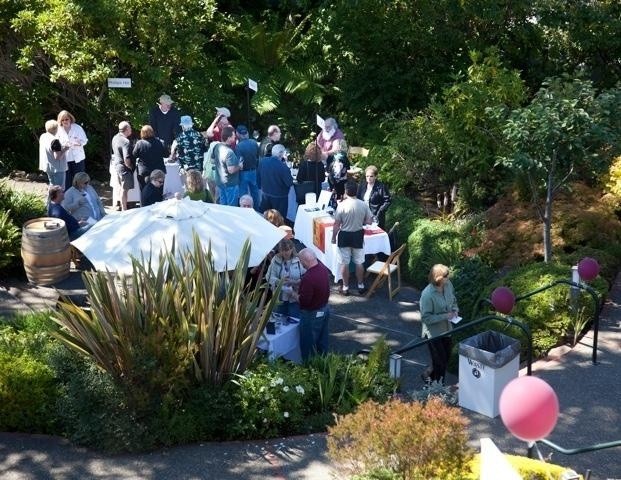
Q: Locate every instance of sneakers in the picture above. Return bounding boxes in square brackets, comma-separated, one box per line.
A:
[338, 285, 367, 295]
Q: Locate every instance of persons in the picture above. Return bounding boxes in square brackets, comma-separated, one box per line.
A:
[39, 110, 106, 271]
[265, 237, 307, 317]
[288, 248, 330, 381]
[111, 95, 391, 296]
[420, 263, 460, 387]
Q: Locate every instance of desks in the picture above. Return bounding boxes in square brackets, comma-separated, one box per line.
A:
[292, 203, 392, 284]
[285, 166, 331, 222]
[257, 312, 302, 364]
[109, 157, 183, 203]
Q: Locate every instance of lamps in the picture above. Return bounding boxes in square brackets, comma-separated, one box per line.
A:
[570, 265, 582, 286]
[389, 353, 403, 380]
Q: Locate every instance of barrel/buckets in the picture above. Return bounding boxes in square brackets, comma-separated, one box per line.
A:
[22, 216, 70, 285]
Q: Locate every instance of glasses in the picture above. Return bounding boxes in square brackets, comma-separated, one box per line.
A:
[365, 175, 375, 179]
[156, 180, 166, 183]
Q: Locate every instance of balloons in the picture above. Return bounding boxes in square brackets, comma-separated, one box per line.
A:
[498, 375, 560, 442]
[578, 257, 600, 280]
[491, 287, 515, 313]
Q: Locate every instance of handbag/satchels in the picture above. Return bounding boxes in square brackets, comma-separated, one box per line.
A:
[294, 180, 314, 203]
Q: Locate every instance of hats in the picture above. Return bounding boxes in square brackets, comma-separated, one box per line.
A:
[179, 116, 195, 126]
[236, 127, 247, 136]
[160, 95, 174, 105]
[215, 106, 230, 116]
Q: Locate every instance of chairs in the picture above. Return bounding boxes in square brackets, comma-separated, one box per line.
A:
[367, 243, 409, 301]
[344, 144, 370, 179]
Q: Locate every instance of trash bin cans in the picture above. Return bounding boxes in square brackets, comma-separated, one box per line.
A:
[458, 330, 521, 419]
